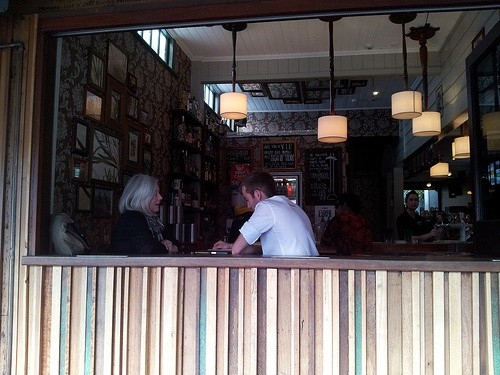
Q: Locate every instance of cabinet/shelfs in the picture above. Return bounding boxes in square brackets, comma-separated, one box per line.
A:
[167, 108, 220, 254]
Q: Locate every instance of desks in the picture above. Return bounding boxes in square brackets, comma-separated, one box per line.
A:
[177, 223, 496, 258]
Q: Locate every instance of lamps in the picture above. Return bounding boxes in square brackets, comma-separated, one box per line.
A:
[430, 144, 449, 178]
[318, 16, 347, 144]
[389, 12, 422, 119]
[451, 121, 470, 159]
[219, 22, 248, 120]
[405, 22, 441, 136]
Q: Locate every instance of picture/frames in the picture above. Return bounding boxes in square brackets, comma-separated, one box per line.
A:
[69, 38, 156, 249]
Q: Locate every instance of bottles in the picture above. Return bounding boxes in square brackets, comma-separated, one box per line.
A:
[420, 206, 459, 224]
[176, 93, 217, 210]
[494, 161, 500, 184]
[488, 163, 495, 185]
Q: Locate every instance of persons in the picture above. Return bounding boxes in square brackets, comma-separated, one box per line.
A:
[212, 170, 320, 257]
[315, 192, 372, 255]
[396, 190, 442, 241]
[111, 173, 181, 256]
